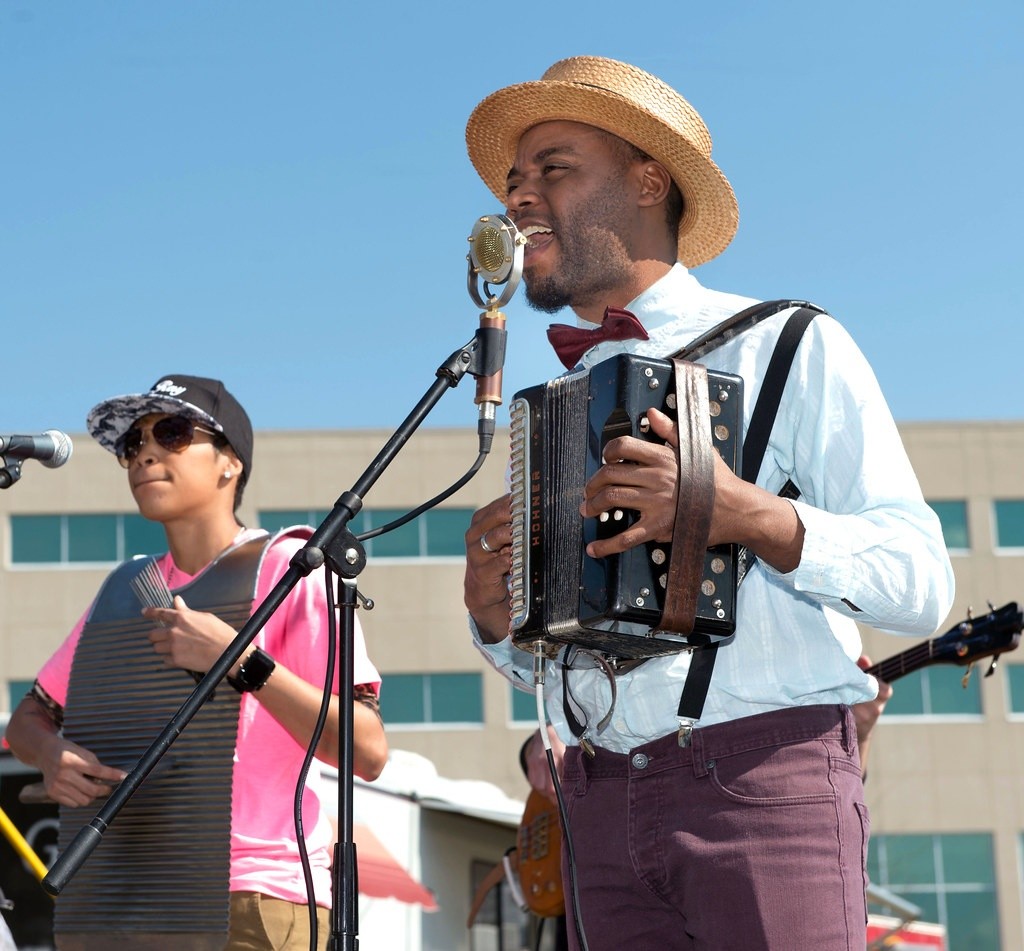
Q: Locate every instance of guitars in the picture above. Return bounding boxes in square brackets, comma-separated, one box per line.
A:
[515, 596, 1023, 923]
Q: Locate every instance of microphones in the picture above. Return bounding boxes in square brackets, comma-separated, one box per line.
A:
[466, 215, 529, 454]
[0, 429, 74, 469]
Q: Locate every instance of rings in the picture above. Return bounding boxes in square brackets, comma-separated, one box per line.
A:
[480, 531, 493, 552]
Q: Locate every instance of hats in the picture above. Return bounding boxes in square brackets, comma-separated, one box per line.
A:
[466, 56, 739, 269]
[86, 374, 252, 464]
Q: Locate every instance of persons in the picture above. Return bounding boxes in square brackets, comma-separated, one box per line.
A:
[6, 373, 388, 951]
[465, 57, 956, 951]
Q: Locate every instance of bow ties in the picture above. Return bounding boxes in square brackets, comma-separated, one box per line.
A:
[545, 306, 649, 372]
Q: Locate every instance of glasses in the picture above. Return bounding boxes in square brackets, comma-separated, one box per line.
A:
[115, 415, 216, 468]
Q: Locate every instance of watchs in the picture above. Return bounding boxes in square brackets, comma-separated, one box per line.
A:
[226, 646, 276, 694]
